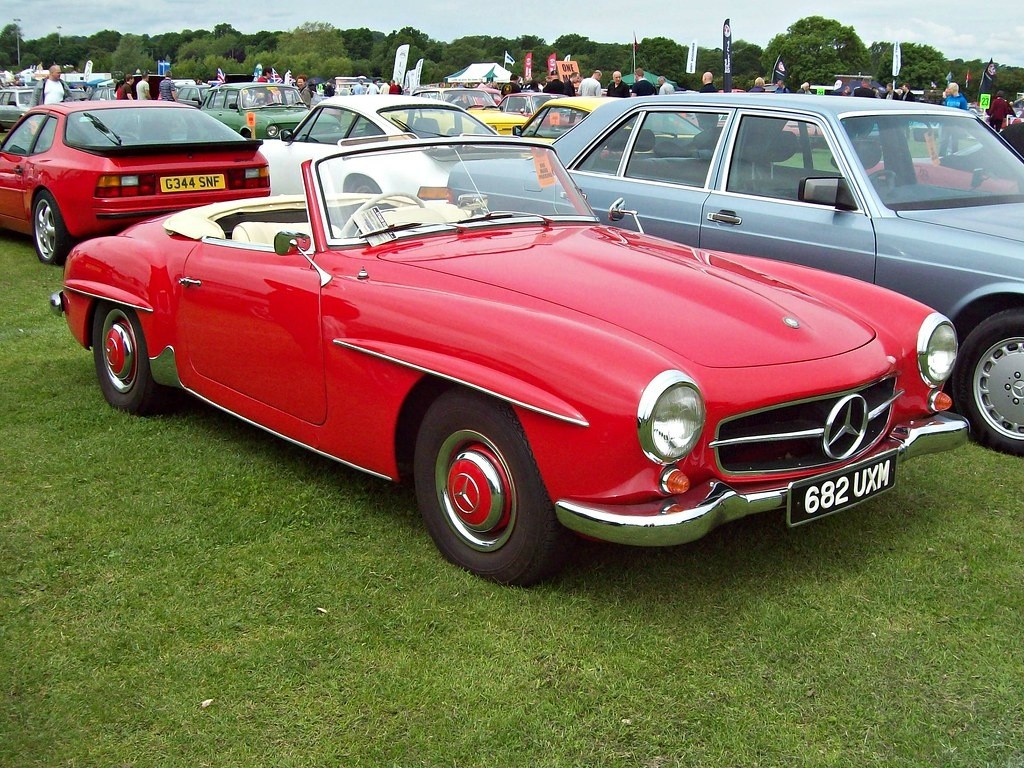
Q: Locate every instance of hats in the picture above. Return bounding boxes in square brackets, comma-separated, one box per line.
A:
[997, 91, 1004, 96]
[359, 79, 363, 83]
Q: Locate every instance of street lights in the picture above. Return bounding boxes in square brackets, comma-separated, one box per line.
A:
[13, 18, 22, 67]
[56, 26, 62, 44]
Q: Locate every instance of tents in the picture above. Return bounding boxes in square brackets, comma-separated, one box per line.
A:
[610, 70, 677, 87]
[443, 62, 512, 83]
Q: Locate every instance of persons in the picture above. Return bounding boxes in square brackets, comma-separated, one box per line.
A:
[499, 70, 601, 100]
[30, 65, 74, 129]
[451, 81, 499, 90]
[14, 75, 25, 86]
[136, 72, 153, 99]
[834, 78, 1016, 156]
[310, 79, 334, 96]
[257, 66, 272, 82]
[297, 75, 311, 106]
[114, 74, 134, 101]
[196, 78, 202, 85]
[748, 77, 812, 94]
[352, 79, 402, 95]
[607, 68, 675, 97]
[159, 71, 177, 101]
[696, 73, 719, 129]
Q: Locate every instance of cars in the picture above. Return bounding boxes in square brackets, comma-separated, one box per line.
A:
[199, 82, 343, 144]
[0, 99, 272, 265]
[469, 87, 583, 128]
[512, 95, 705, 154]
[908, 88, 1024, 141]
[0, 72, 256, 132]
[258, 94, 501, 203]
[352, 86, 536, 137]
[674, 83, 836, 152]
[444, 91, 1023, 459]
[866, 120, 1023, 196]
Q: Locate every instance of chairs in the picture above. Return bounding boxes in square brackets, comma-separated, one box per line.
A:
[413, 118, 442, 138]
[596, 125, 896, 210]
[361, 124, 384, 136]
[382, 201, 466, 228]
[232, 222, 344, 243]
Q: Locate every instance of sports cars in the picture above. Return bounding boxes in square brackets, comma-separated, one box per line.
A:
[47, 134, 971, 588]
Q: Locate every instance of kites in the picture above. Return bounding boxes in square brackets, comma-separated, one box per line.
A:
[208, 68, 226, 85]
[252, 64, 263, 82]
[270, 67, 296, 85]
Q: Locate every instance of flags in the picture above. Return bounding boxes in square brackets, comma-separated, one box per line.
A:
[946, 58, 995, 95]
[635, 37, 638, 55]
[506, 53, 515, 65]
